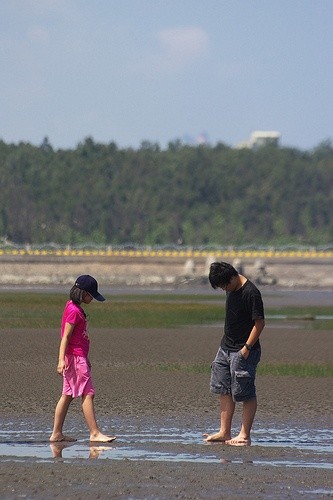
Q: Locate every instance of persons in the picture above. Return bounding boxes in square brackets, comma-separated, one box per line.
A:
[50, 275, 116, 441]
[203, 261, 265, 444]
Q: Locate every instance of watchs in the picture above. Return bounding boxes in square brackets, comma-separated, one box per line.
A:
[244, 343, 253, 350]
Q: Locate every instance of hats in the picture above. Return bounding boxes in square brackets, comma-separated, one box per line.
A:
[75, 275, 105, 301]
